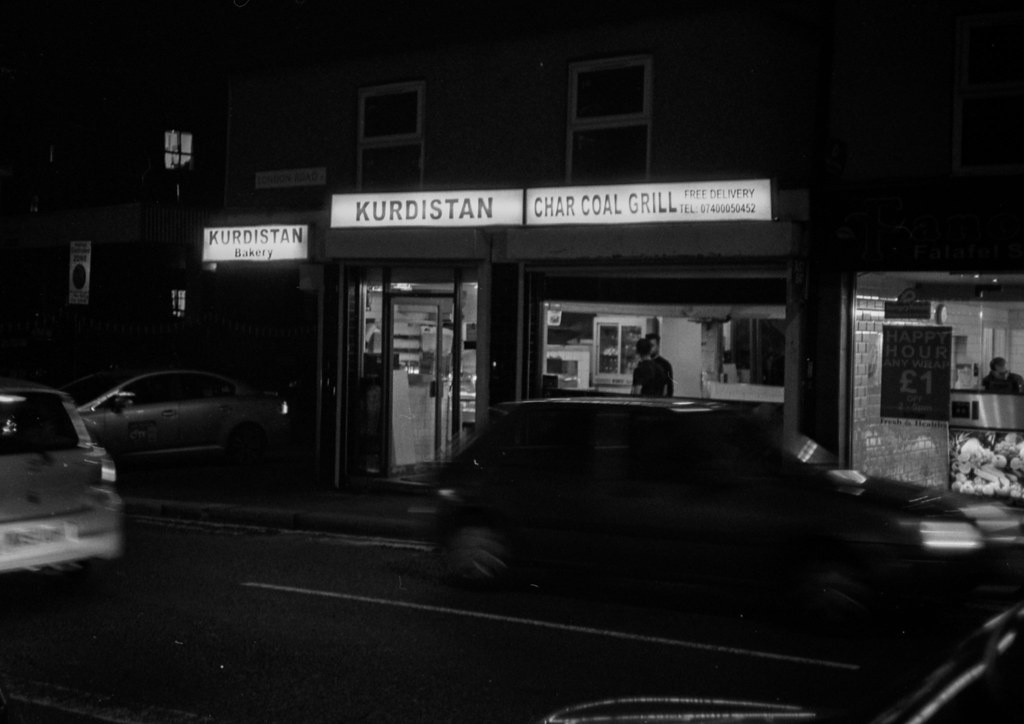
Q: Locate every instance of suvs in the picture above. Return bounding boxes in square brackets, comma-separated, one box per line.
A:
[429, 389, 1023, 642]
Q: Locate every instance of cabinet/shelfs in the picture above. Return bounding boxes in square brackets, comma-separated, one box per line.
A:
[591, 315, 658, 387]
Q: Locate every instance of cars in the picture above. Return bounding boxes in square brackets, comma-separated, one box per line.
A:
[0, 382, 133, 589]
[60, 365, 289, 484]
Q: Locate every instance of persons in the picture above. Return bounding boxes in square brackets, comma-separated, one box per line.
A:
[629, 331, 673, 398]
[982, 356, 1023, 394]
